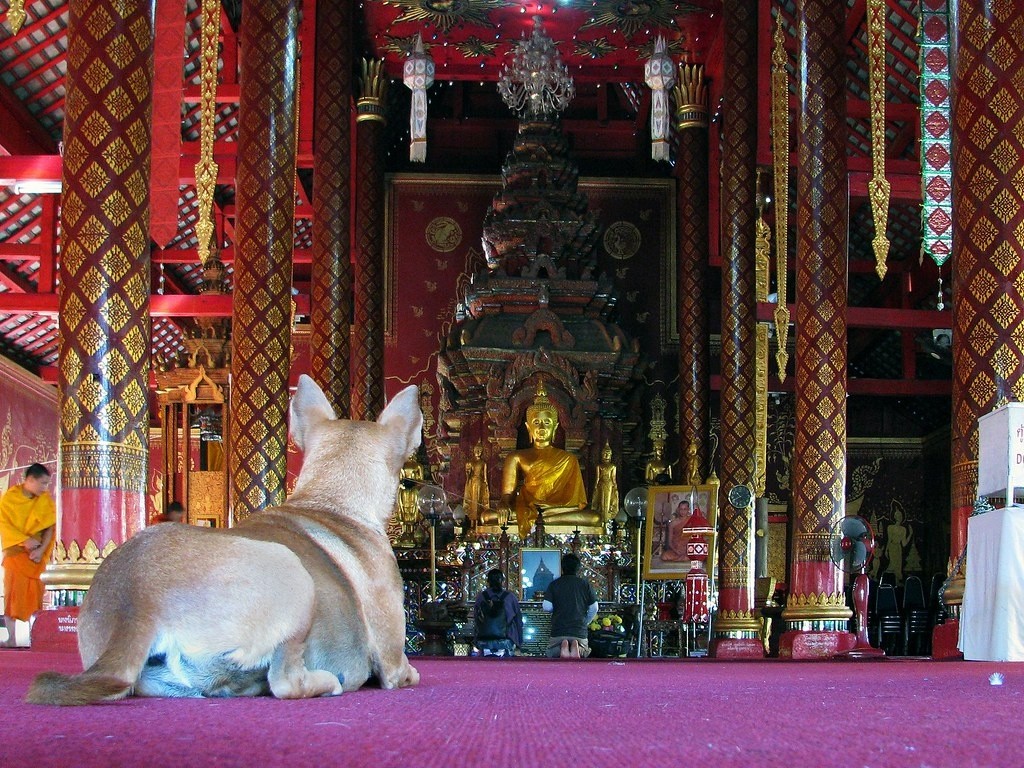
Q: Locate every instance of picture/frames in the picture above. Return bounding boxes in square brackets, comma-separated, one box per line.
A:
[519, 548, 563, 602]
[643, 484, 718, 580]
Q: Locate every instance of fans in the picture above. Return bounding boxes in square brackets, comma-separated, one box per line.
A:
[829, 515, 889, 664]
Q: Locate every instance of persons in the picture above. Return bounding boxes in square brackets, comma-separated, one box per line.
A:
[661, 501, 692, 561]
[151, 501, 184, 525]
[0, 463, 56, 648]
[542, 553, 600, 659]
[397, 450, 422, 543]
[473, 568, 523, 658]
[480, 377, 602, 525]
[591, 437, 620, 521]
[461, 438, 491, 530]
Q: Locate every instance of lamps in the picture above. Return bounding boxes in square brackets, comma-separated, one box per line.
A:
[497, 15, 575, 117]
[682, 485, 715, 625]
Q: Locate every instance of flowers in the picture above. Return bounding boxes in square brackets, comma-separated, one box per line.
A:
[589, 614, 625, 632]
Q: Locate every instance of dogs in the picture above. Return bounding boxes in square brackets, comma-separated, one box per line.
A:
[27, 374, 423, 706]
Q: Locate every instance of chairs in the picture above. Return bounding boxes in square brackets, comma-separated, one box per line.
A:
[845, 572, 946, 656]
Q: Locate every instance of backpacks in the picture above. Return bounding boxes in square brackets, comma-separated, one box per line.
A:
[477, 591, 514, 639]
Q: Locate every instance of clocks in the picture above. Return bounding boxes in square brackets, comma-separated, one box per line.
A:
[728, 485, 752, 508]
